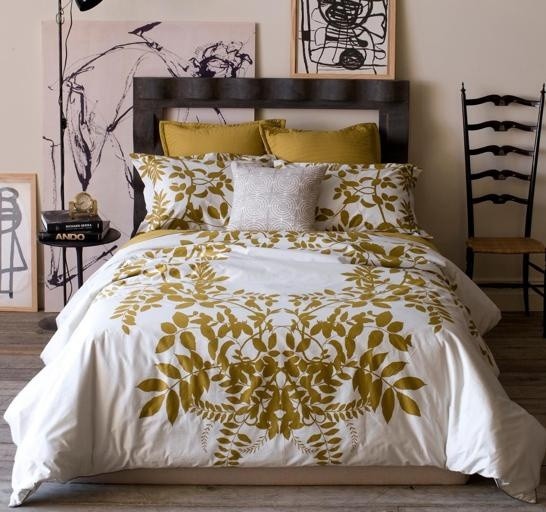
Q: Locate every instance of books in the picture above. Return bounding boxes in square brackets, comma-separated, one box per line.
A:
[38, 210, 104, 234]
[39, 220, 112, 241]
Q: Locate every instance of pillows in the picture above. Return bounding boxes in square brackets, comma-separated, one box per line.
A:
[128, 119, 423, 236]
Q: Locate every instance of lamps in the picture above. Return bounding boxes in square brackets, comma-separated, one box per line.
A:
[57, 0, 102, 312]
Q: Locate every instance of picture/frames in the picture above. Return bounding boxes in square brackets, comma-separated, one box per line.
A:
[0, 173, 40, 314]
[289, 0, 397, 79]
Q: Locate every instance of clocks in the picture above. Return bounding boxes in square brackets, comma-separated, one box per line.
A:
[68, 192, 98, 218]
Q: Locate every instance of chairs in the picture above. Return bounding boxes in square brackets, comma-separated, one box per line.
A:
[459, 81, 546, 337]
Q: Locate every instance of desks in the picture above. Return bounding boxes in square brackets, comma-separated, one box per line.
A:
[37, 228, 123, 332]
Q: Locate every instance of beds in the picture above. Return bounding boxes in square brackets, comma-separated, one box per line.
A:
[52, 75, 493, 486]
